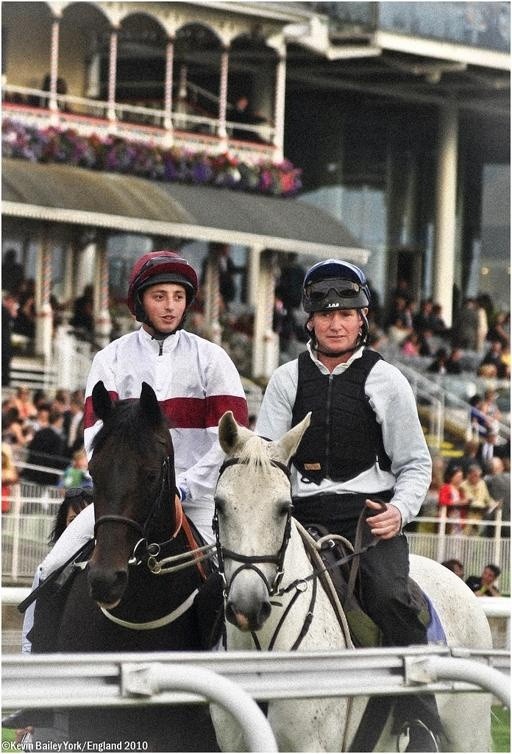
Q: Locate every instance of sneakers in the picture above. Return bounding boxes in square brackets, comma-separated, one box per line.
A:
[2, 708, 55, 730]
[404, 718, 449, 752]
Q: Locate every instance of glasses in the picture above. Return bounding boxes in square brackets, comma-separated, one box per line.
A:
[302, 277, 361, 301]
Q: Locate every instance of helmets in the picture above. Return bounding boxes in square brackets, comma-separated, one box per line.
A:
[302, 258, 370, 313]
[127, 249, 199, 315]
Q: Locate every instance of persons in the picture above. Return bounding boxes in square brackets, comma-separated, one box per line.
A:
[2, 250, 250, 729]
[2, 243, 510, 601]
[253, 258, 452, 752]
[229, 94, 265, 139]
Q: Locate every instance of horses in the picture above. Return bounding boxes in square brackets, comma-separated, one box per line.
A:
[32, 378, 224, 752]
[207, 410, 495, 753]
